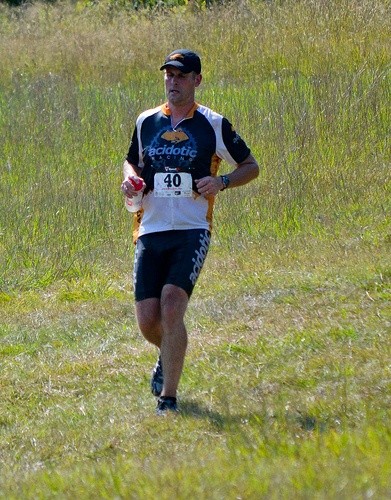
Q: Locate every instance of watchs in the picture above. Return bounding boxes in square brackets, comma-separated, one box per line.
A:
[220, 175, 230, 191]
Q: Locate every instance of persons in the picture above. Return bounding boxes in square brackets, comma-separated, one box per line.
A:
[119, 49, 260, 413]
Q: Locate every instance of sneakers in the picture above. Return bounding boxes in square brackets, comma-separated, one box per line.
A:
[156, 396, 177, 417]
[149, 356, 164, 395]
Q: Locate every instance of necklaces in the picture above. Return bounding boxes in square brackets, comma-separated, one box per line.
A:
[169, 101, 195, 128]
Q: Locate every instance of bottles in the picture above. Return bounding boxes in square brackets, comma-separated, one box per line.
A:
[125, 179, 143, 212]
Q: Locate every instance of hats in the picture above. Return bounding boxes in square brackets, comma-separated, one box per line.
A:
[160, 49, 201, 75]
